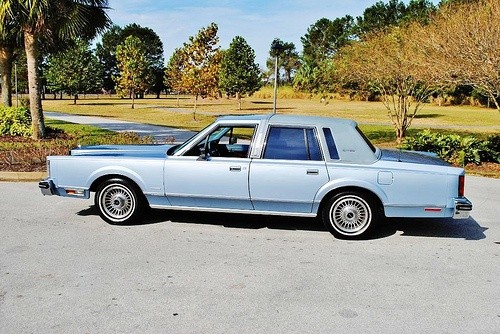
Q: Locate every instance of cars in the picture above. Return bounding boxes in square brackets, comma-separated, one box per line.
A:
[42, 111, 473, 239]
